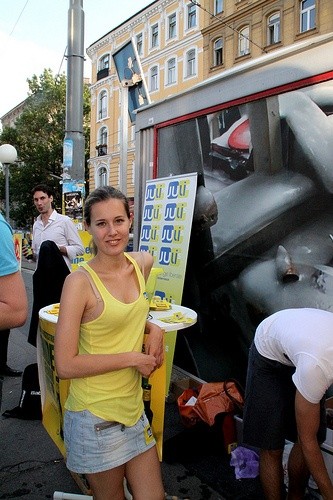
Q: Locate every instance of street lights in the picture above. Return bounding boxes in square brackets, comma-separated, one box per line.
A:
[0, 144, 17, 222]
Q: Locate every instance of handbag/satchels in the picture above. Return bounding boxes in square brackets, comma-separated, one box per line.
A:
[2, 363, 41, 420]
[177, 382, 243, 455]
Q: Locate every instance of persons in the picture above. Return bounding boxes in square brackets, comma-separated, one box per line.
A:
[242, 308, 333, 500]
[0, 213, 28, 375]
[54, 186, 164, 500]
[22, 184, 85, 272]
